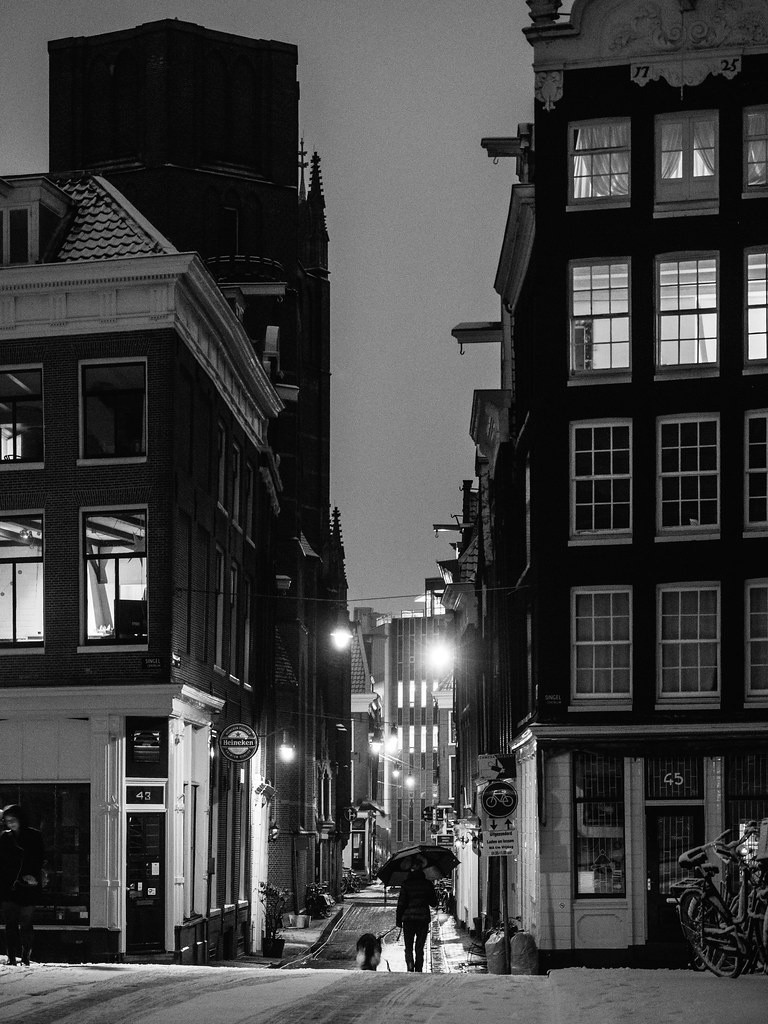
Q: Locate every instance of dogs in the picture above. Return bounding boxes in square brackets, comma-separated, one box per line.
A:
[356, 933, 381, 971]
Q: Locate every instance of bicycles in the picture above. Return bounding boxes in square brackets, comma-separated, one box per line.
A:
[669, 819, 768, 978]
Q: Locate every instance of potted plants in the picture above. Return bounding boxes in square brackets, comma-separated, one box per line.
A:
[253, 880, 292, 957]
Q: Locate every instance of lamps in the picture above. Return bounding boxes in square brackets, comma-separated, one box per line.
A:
[488, 758, 505, 774]
[18, 528, 33, 539]
[257, 725, 298, 765]
[268, 821, 279, 844]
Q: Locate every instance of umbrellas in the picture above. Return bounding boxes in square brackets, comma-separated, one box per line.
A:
[377, 844, 462, 887]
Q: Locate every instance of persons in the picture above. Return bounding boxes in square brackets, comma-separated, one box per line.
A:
[0, 804, 42, 966]
[394, 859, 438, 973]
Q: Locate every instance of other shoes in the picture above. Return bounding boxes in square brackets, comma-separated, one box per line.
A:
[3, 957, 17, 966]
[16, 958, 30, 966]
